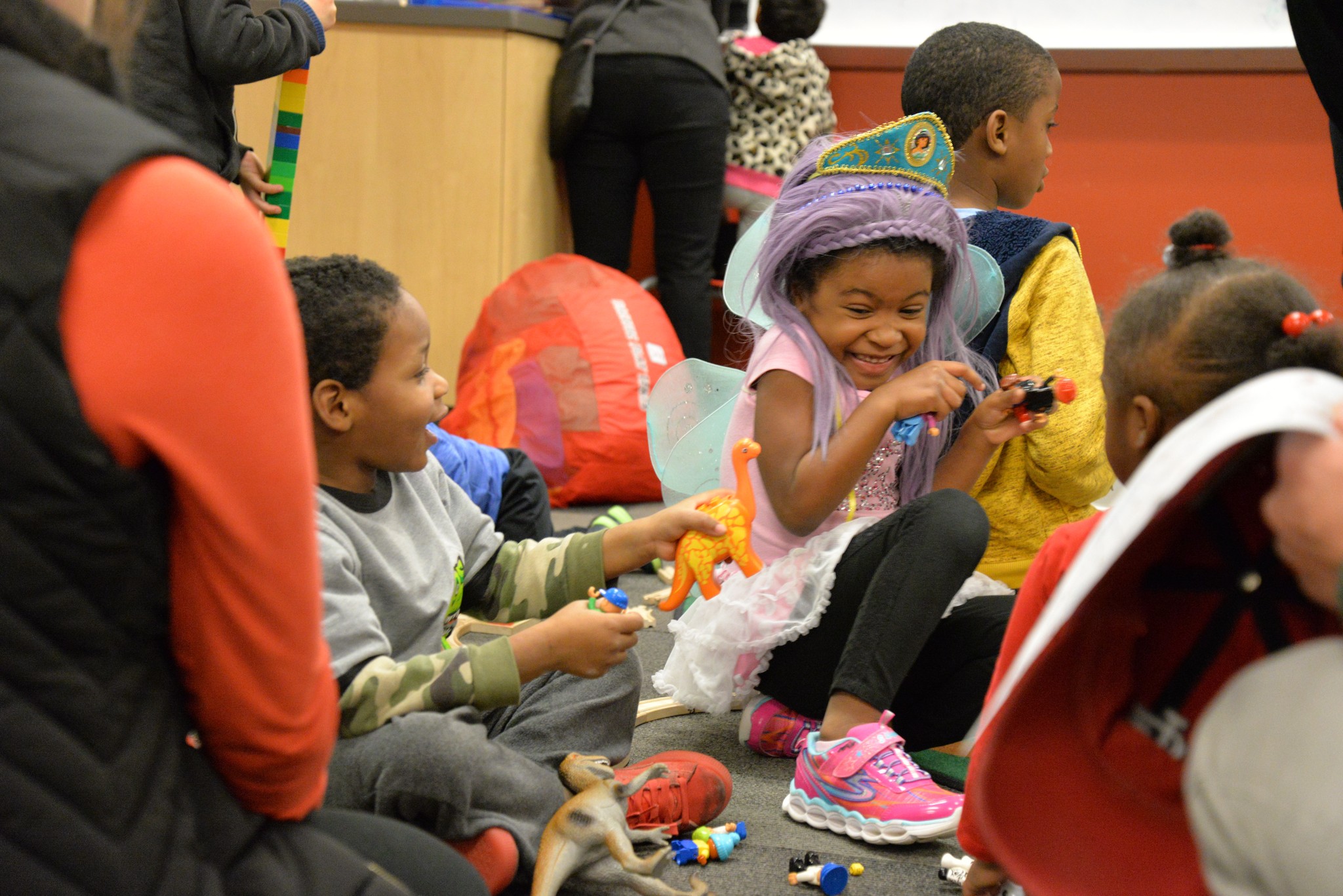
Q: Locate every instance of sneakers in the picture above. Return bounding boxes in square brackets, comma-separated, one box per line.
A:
[613, 750, 732, 840]
[440, 827, 519, 895]
[781, 710, 965, 847]
[737, 691, 824, 760]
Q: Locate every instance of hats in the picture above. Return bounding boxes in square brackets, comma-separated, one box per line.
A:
[976, 362, 1343, 892]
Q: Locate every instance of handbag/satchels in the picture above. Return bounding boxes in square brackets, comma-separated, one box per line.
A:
[548, 34, 598, 163]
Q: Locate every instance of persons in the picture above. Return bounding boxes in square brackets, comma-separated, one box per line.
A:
[112, 0, 342, 222]
[651, 132, 1024, 850]
[413, 409, 665, 578]
[1179, 390, 1342, 896]
[530, 0, 832, 365]
[267, 244, 735, 896]
[889, 16, 1116, 600]
[957, 212, 1342, 896]
[0, 0, 499, 896]
